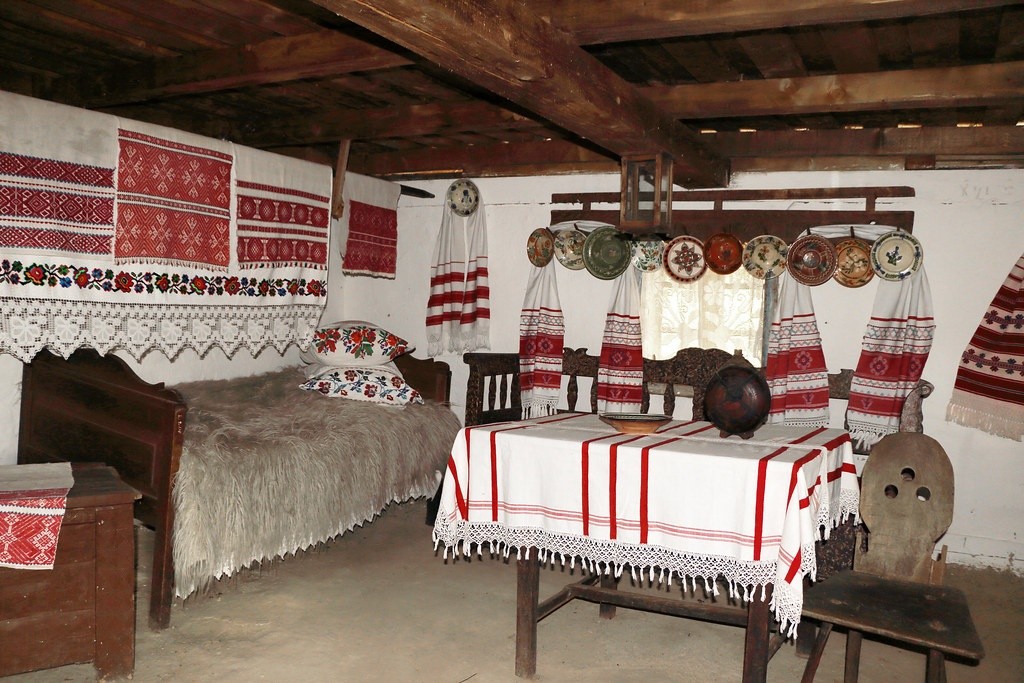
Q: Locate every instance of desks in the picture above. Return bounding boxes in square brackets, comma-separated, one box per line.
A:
[430, 411, 859, 683]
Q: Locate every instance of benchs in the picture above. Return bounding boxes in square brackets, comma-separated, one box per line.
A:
[464, 346, 936, 580]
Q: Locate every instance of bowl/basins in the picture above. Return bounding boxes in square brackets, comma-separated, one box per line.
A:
[599, 412, 673, 435]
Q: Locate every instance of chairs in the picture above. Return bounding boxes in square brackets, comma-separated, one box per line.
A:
[804, 431, 986, 683]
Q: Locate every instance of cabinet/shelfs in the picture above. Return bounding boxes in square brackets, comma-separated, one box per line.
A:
[0, 462, 144, 682]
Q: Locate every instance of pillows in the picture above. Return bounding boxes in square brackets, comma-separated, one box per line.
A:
[297, 360, 425, 406]
[299, 320, 416, 366]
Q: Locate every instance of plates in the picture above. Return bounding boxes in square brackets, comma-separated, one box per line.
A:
[832, 238, 875, 288]
[554, 229, 588, 271]
[582, 225, 632, 280]
[870, 231, 923, 282]
[741, 235, 789, 280]
[446, 178, 480, 217]
[526, 228, 554, 268]
[786, 234, 838, 287]
[703, 232, 744, 275]
[662, 235, 706, 284]
[629, 232, 665, 273]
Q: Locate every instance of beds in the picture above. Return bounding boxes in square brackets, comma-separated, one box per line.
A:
[17, 349, 461, 632]
